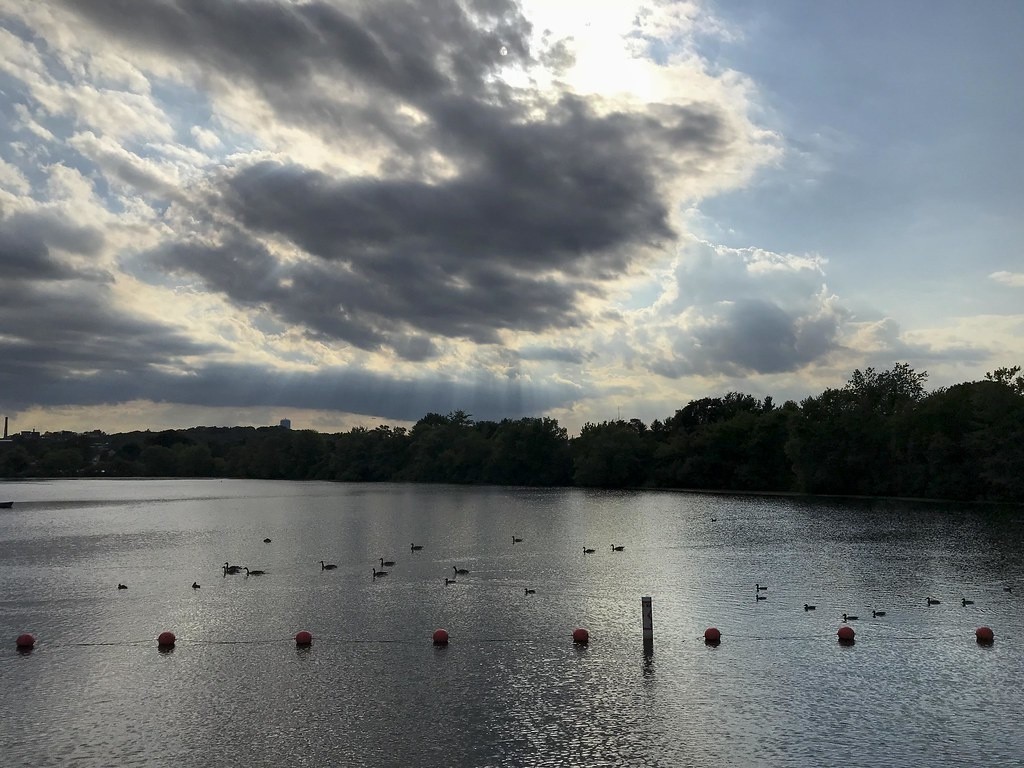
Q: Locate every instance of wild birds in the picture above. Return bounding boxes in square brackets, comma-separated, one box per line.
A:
[410, 543, 423, 550]
[453, 566, 469, 574]
[192, 582, 200, 588]
[118, 584, 127, 589]
[804, 604, 816, 610]
[755, 584, 768, 600]
[524, 588, 534, 592]
[582, 544, 623, 553]
[319, 561, 337, 571]
[925, 597, 974, 604]
[710, 518, 717, 522]
[264, 538, 270, 542]
[872, 610, 886, 616]
[372, 568, 388, 576]
[222, 562, 264, 574]
[445, 578, 455, 583]
[843, 614, 858, 620]
[512, 536, 524, 542]
[379, 558, 395, 565]
